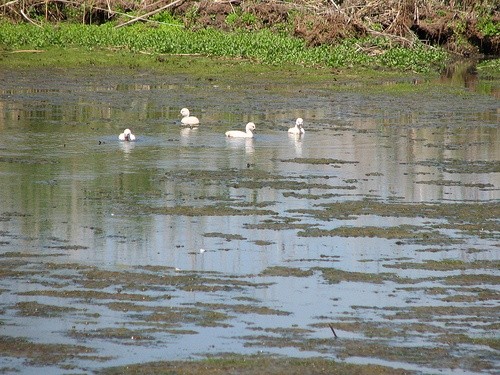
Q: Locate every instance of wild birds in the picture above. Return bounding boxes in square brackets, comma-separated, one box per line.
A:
[178, 107, 199, 124]
[288, 118, 305, 134]
[225, 121, 257, 138]
[119, 128, 136, 141]
